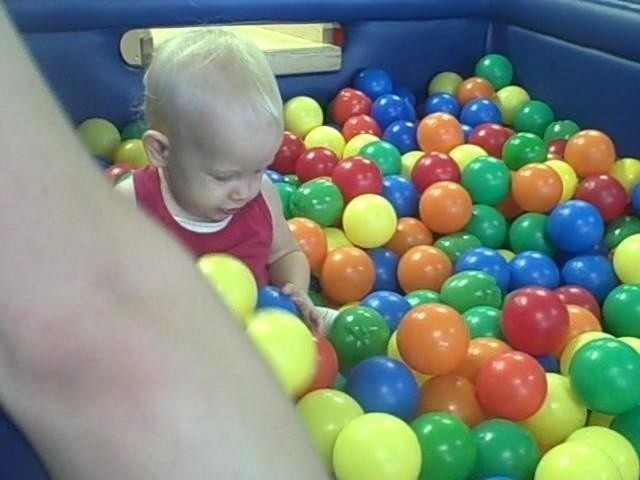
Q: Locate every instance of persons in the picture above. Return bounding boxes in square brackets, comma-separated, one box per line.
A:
[1, 0, 333, 480]
[112, 28, 324, 342]
